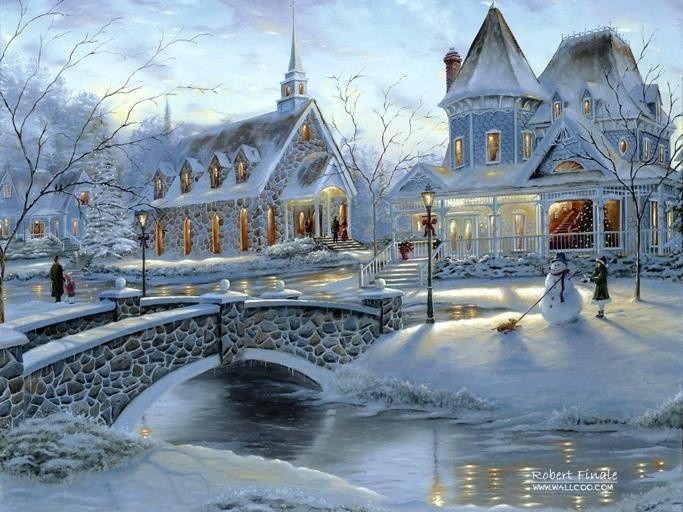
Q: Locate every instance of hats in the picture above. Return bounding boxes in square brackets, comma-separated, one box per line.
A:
[596, 255, 607, 266]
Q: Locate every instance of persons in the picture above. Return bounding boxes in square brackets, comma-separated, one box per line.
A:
[582, 256, 611, 317]
[304, 216, 313, 238]
[330, 215, 349, 243]
[49, 256, 77, 304]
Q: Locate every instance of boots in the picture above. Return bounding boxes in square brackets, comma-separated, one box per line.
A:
[595, 310, 604, 317]
